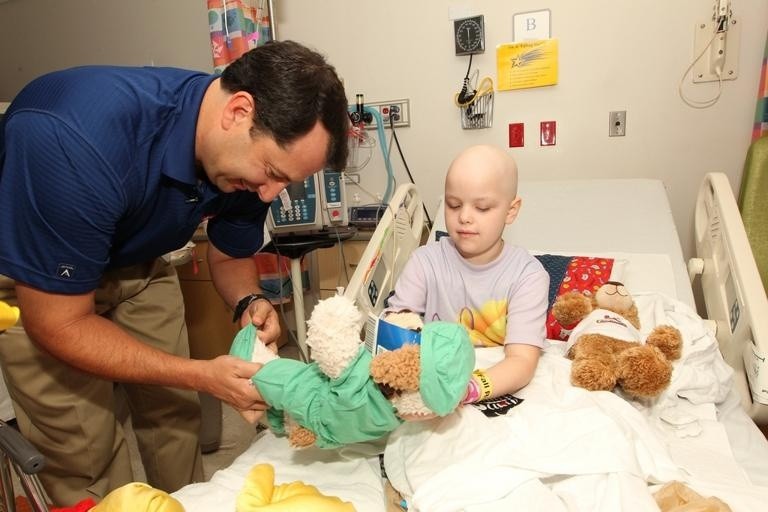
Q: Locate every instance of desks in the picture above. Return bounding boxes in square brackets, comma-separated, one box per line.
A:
[260, 225, 355, 362]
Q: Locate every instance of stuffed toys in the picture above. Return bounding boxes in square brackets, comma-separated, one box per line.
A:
[552, 281, 684, 400]
[226, 292, 476, 451]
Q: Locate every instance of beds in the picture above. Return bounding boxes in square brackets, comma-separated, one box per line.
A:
[72, 169, 767, 512]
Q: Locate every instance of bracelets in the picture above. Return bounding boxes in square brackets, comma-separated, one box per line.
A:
[462, 381, 480, 404]
[232, 293, 270, 324]
[473, 369, 493, 403]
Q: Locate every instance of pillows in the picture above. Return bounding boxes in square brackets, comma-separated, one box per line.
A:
[436, 229, 680, 342]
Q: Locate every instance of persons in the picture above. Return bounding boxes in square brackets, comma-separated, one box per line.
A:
[0, 39, 352, 510]
[384, 141, 553, 405]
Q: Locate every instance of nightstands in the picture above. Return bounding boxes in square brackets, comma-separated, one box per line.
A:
[316, 218, 435, 312]
